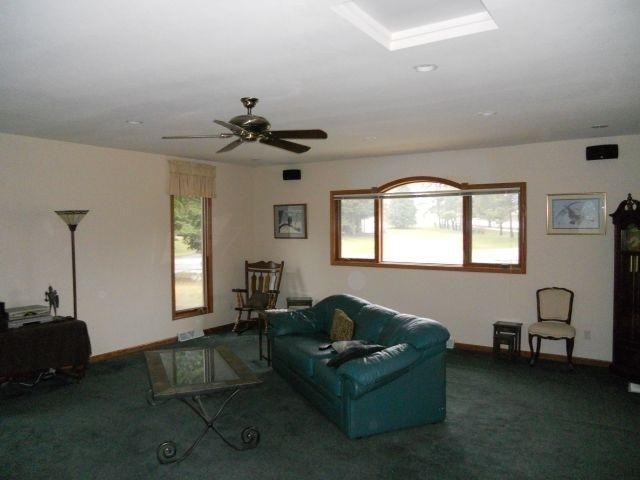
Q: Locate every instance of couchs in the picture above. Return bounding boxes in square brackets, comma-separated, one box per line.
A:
[264, 294, 450, 441]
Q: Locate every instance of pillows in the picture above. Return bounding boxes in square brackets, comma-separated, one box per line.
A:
[329, 309, 386, 354]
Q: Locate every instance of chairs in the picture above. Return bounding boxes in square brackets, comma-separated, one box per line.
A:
[529, 287, 576, 370]
[231, 259, 284, 332]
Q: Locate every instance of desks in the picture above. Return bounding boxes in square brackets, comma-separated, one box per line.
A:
[143, 345, 264, 465]
[492, 321, 522, 363]
[286, 297, 313, 310]
[1, 317, 84, 386]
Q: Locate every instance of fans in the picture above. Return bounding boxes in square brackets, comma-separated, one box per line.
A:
[162, 96, 327, 155]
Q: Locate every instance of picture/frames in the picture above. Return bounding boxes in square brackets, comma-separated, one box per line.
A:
[547, 193, 608, 235]
[274, 204, 308, 238]
